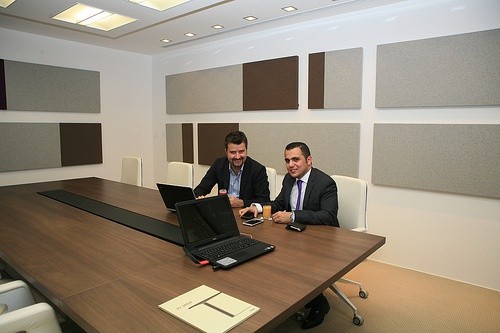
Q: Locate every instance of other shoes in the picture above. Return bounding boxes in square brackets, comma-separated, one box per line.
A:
[301, 300, 331, 330]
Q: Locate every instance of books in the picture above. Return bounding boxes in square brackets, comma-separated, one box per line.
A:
[158, 284, 260, 333]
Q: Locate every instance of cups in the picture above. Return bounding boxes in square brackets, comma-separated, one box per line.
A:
[263, 203, 271, 219]
[219, 189, 227, 196]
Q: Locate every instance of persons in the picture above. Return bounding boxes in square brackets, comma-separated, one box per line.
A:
[193, 131, 271, 207]
[238, 141, 340, 329]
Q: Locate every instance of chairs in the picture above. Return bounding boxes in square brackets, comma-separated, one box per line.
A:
[0, 266, 67, 333]
[121, 157, 369, 327]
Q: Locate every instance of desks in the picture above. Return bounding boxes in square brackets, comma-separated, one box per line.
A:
[0, 176, 386, 333]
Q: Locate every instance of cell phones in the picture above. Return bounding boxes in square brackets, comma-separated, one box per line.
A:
[242, 218, 264, 227]
[241, 212, 254, 219]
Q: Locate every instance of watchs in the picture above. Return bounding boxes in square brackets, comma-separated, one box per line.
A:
[289, 212, 293, 220]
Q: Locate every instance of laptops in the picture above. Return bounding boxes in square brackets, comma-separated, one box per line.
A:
[156, 183, 196, 211]
[175, 194, 276, 270]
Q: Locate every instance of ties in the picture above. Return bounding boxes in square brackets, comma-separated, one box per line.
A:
[295, 180, 304, 210]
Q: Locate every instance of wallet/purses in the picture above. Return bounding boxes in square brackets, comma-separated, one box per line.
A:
[285, 221, 306, 232]
